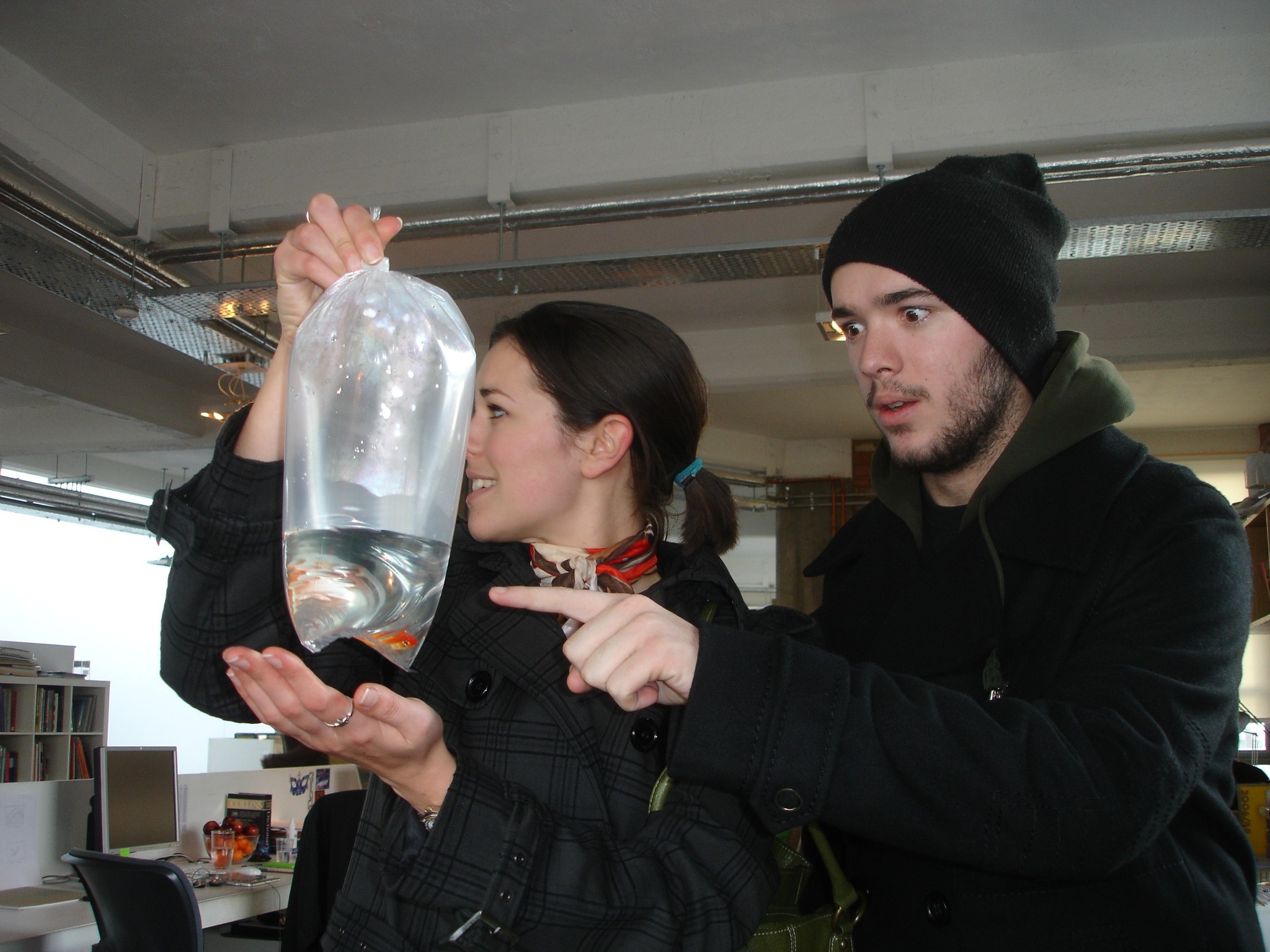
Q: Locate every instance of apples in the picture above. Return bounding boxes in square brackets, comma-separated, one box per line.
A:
[202, 816, 260, 837]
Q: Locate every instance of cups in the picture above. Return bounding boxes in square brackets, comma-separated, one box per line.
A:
[211, 829, 235, 873]
[276, 838, 293, 862]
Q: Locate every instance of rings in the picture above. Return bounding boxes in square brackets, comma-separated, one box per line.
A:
[306, 210, 311, 224]
[325, 696, 355, 727]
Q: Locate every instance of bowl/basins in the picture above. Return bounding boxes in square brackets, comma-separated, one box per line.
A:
[203, 834, 259, 864]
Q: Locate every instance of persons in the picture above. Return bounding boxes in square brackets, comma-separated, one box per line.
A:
[148, 192, 747, 952]
[488, 153, 1266, 952]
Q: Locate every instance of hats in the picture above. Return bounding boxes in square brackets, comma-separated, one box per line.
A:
[822, 153, 1069, 400]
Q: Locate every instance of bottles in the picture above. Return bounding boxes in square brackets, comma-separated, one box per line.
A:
[286, 817, 297, 853]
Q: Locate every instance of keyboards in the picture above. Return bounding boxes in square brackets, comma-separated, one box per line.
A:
[181, 864, 228, 882]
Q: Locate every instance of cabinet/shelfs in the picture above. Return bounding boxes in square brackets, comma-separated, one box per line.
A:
[0, 673, 111, 783]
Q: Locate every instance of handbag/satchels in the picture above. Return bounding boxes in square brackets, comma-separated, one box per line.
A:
[648, 600, 868, 952]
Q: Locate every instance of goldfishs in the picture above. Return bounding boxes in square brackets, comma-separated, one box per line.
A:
[286, 554, 418, 653]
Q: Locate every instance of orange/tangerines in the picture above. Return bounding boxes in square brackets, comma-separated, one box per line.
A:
[204, 833, 254, 865]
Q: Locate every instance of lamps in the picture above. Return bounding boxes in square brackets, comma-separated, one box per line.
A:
[199, 348, 273, 422]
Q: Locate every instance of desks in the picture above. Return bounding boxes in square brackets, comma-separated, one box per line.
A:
[0, 853, 294, 952]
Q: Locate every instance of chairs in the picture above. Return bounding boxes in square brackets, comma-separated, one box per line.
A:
[63, 847, 285, 950]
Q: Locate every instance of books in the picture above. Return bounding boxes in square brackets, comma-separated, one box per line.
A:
[34, 687, 64, 733]
[254, 861, 295, 873]
[0, 646, 88, 679]
[33, 742, 44, 781]
[69, 735, 88, 779]
[226, 792, 273, 856]
[0, 688, 18, 732]
[0, 745, 19, 783]
[71, 693, 98, 733]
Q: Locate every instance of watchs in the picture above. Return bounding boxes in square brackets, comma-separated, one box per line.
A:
[418, 805, 442, 832]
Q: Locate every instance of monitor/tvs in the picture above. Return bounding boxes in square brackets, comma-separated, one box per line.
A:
[86, 746, 179, 854]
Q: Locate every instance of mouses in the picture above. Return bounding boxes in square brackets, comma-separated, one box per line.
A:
[234, 867, 261, 876]
